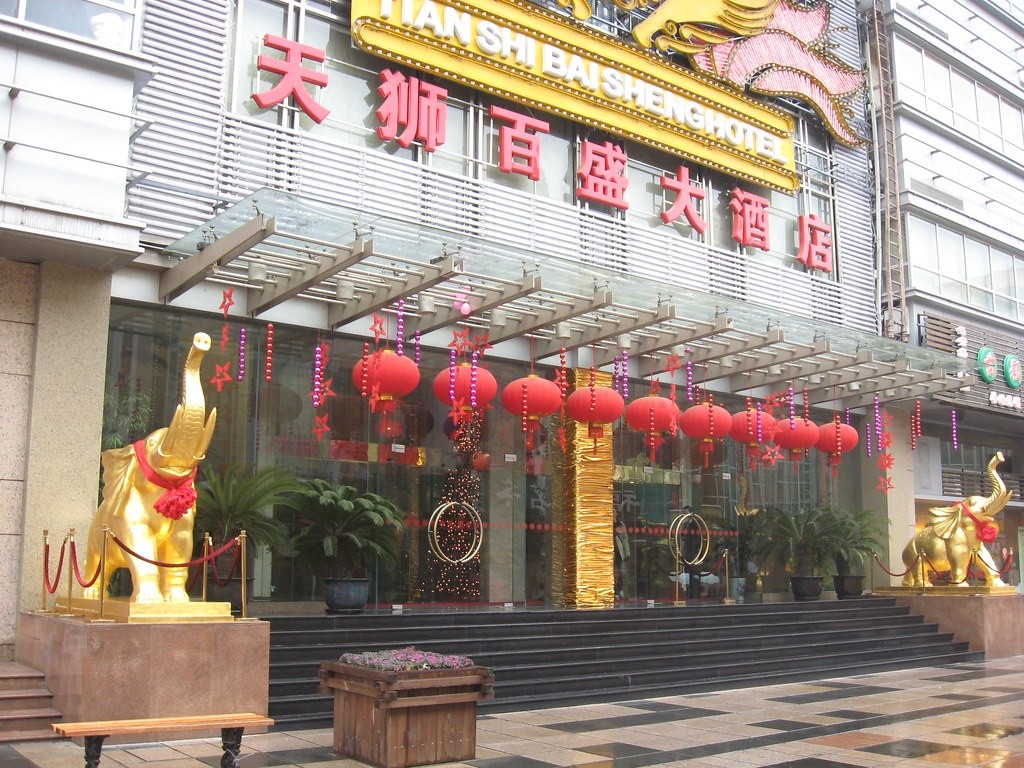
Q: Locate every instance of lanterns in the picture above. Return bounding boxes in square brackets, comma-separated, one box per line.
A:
[814, 419, 859, 479]
[502, 374, 562, 449]
[728, 406, 778, 469]
[679, 402, 732, 468]
[432, 362, 498, 426]
[566, 386, 624, 456]
[352, 351, 421, 433]
[625, 393, 680, 462]
[773, 415, 819, 476]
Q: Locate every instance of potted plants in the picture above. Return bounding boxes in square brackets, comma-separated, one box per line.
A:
[195, 459, 321, 617]
[293, 478, 406, 615]
[707, 514, 752, 604]
[831, 506, 894, 600]
[749, 506, 836, 602]
[759, 539, 793, 593]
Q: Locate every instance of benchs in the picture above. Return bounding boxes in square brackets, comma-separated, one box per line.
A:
[50, 712, 274, 768]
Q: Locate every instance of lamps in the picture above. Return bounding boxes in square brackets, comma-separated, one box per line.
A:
[768, 365, 781, 377]
[883, 389, 895, 398]
[847, 383, 859, 392]
[671, 345, 685, 359]
[719, 356, 732, 369]
[556, 322, 571, 340]
[808, 374, 821, 385]
[418, 295, 435, 315]
[248, 262, 266, 284]
[490, 309, 506, 328]
[336, 279, 354, 300]
[617, 333, 631, 349]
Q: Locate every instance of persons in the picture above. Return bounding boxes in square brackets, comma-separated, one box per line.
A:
[612, 507, 632, 601]
[680, 505, 703, 599]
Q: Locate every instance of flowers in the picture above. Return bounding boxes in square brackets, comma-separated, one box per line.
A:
[338, 645, 473, 672]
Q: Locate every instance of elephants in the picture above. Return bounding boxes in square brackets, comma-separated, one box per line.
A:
[902, 452, 1013, 586]
[83, 333, 218, 604]
